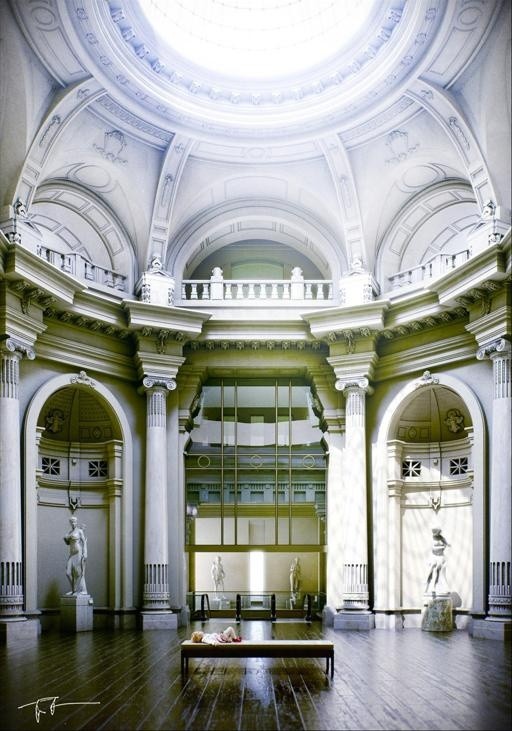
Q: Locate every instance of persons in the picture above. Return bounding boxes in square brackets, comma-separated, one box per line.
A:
[211, 557, 227, 600]
[191, 626, 241, 647]
[63, 517, 87, 595]
[289, 557, 300, 599]
[424, 529, 451, 593]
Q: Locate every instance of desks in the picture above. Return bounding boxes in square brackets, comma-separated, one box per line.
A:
[180, 639, 334, 681]
